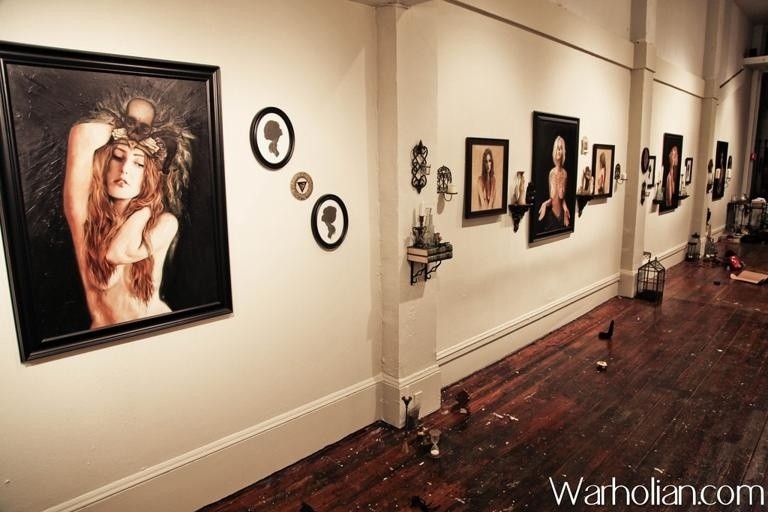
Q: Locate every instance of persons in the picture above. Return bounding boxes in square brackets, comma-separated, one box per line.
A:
[596, 153, 606, 195]
[537, 136, 570, 227]
[477, 149, 497, 208]
[62, 123, 179, 328]
[664, 146, 678, 208]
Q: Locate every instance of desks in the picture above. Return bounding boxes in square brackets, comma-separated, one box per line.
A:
[727, 199, 768, 234]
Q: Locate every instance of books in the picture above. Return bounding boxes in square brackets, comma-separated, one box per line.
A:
[408, 243, 452, 255]
[405, 254, 452, 265]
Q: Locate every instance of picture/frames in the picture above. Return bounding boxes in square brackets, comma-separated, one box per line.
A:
[0, 41, 733, 367]
